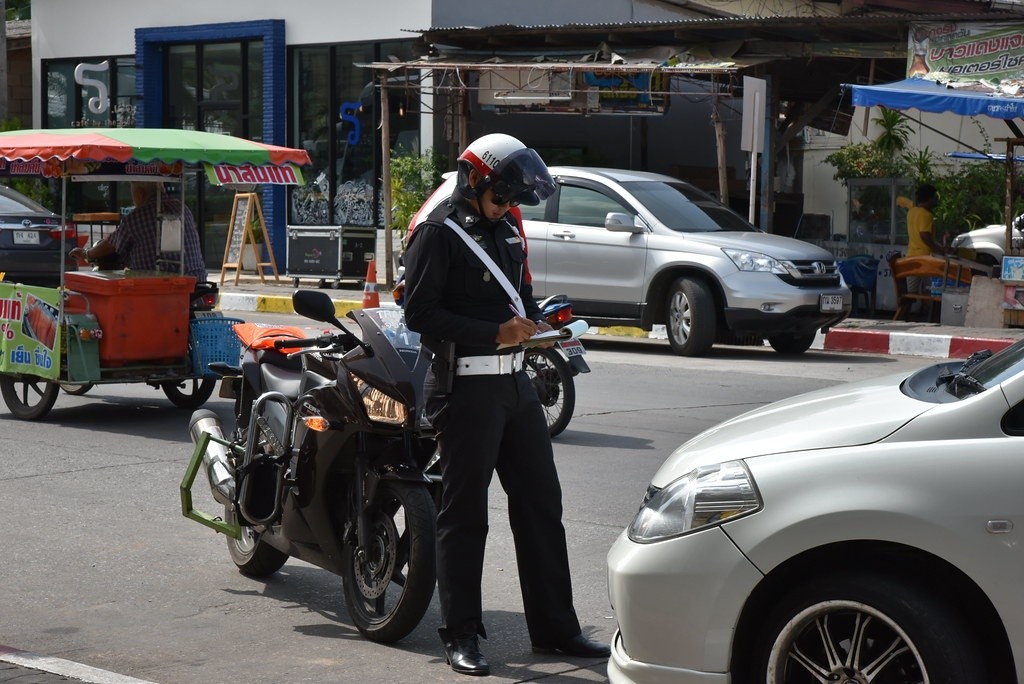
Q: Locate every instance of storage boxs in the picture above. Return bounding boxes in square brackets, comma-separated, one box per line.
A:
[64, 267, 198, 367]
[285, 225, 378, 289]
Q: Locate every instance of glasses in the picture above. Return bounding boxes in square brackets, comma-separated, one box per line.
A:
[486, 183, 520, 207]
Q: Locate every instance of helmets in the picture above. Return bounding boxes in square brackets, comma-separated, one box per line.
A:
[457, 133, 540, 206]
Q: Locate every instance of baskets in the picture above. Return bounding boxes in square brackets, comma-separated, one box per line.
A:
[188, 315, 245, 381]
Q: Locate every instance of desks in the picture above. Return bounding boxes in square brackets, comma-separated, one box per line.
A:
[73, 213, 121, 248]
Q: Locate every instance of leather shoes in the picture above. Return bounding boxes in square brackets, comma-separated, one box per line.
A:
[444, 633, 491, 674]
[532, 634, 611, 659]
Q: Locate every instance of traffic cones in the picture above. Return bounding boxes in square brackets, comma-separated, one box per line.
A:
[361, 261, 380, 308]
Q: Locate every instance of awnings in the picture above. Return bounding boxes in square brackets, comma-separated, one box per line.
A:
[843, 77, 1023, 120]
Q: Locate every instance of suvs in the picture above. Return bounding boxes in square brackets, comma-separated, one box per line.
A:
[406, 166, 853, 355]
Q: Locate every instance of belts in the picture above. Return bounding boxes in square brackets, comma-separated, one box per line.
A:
[455, 350, 525, 375]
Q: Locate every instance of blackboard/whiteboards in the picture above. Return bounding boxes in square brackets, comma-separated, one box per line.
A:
[222, 193, 252, 267]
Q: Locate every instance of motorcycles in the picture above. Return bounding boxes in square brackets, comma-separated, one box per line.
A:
[517, 293, 591, 434]
[0, 128, 312, 418]
[180, 289, 442, 641]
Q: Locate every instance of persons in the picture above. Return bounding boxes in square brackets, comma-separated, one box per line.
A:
[893, 185, 942, 321]
[403, 131, 611, 678]
[68, 168, 210, 284]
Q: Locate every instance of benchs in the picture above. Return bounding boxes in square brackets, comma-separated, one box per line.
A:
[886, 249, 1003, 324]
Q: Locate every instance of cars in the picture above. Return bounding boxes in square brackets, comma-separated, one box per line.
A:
[951, 214, 1024, 267]
[606, 341, 1023, 684]
[0, 185, 79, 276]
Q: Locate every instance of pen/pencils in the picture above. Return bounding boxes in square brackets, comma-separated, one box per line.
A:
[509, 304, 538, 336]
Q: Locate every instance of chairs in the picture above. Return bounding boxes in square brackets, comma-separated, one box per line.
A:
[838, 255, 881, 320]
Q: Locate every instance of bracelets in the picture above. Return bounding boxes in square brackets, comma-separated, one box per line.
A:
[83, 249, 87, 258]
